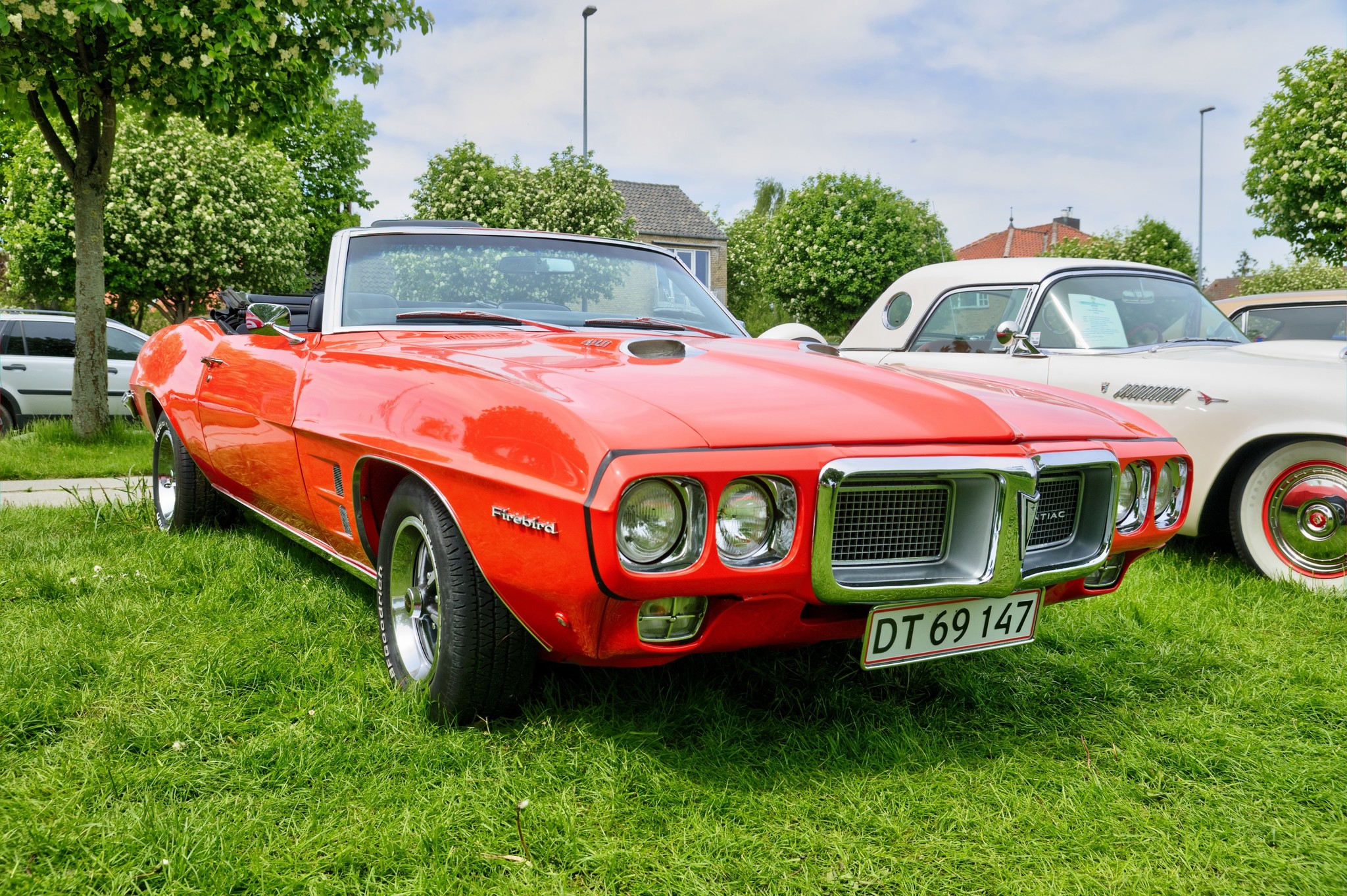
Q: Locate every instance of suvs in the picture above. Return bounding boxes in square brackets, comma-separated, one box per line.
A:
[0, 308, 156, 440]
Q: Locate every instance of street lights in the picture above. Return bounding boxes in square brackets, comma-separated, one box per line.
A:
[579, 5, 600, 166]
[1196, 106, 1215, 292]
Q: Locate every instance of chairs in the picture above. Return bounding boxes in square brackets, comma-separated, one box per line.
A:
[307, 292, 399, 332]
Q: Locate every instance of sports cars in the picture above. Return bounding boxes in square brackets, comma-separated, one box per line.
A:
[123, 216, 1194, 730]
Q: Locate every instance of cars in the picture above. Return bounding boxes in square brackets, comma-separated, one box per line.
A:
[1149, 286, 1347, 355]
[754, 256, 1347, 601]
